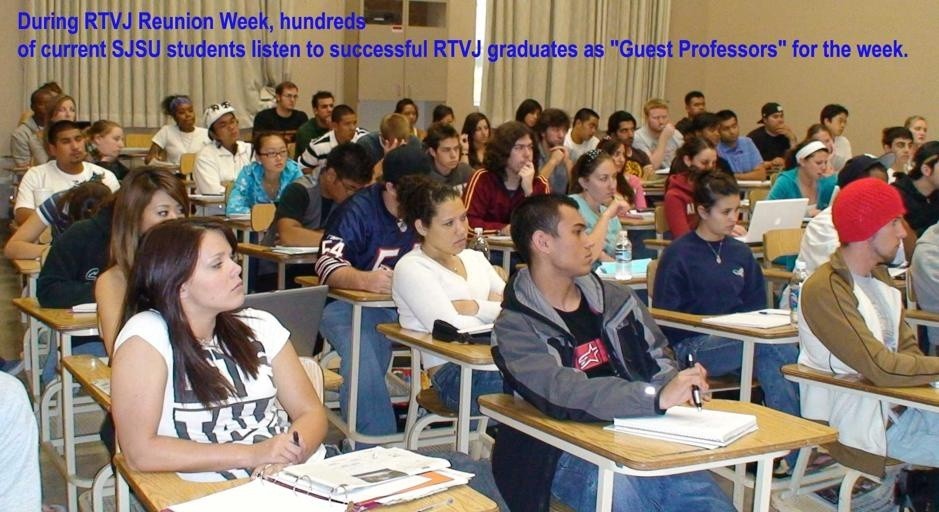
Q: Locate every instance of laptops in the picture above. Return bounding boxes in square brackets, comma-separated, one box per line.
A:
[732, 197, 810, 244]
[230, 284, 329, 358]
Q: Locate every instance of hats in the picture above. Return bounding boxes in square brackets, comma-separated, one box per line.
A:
[204, 100, 236, 142]
[912, 141, 939, 168]
[756, 101, 784, 124]
[830, 176, 907, 246]
[836, 150, 898, 189]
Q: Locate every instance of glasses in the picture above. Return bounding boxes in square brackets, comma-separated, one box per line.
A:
[259, 147, 288, 159]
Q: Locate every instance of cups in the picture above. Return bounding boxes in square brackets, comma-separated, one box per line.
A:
[31, 187, 55, 210]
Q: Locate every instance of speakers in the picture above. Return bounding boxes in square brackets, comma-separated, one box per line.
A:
[408, 0, 446, 28]
[363, 0, 402, 26]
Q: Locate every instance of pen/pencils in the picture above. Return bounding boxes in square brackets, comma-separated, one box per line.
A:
[759, 311, 790, 316]
[685, 354, 705, 409]
[379, 264, 388, 271]
[611, 195, 633, 215]
[294, 431, 298, 446]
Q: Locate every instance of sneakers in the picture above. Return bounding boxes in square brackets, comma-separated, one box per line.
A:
[0, 358, 25, 378]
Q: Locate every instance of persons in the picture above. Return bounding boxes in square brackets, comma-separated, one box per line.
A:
[0, 80, 939, 512]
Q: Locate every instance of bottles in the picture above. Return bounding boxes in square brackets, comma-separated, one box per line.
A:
[468, 225, 492, 262]
[614, 230, 634, 280]
[789, 261, 810, 327]
